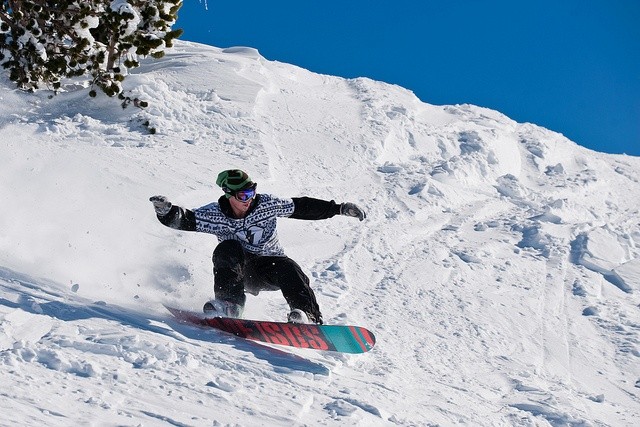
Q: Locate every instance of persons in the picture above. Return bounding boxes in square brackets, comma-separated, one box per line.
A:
[149, 168, 367, 323]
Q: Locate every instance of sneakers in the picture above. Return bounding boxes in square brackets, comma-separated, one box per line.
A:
[203, 300, 241, 319]
[288, 309, 322, 325]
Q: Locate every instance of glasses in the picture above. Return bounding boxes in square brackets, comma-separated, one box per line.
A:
[221, 181, 258, 204]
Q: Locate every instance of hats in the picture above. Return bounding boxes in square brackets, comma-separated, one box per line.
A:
[216, 169, 253, 199]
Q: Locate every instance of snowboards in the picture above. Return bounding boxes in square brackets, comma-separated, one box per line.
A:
[162, 301, 376, 353]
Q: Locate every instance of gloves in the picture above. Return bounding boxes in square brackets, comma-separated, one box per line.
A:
[341, 202, 367, 222]
[149, 194, 172, 215]
